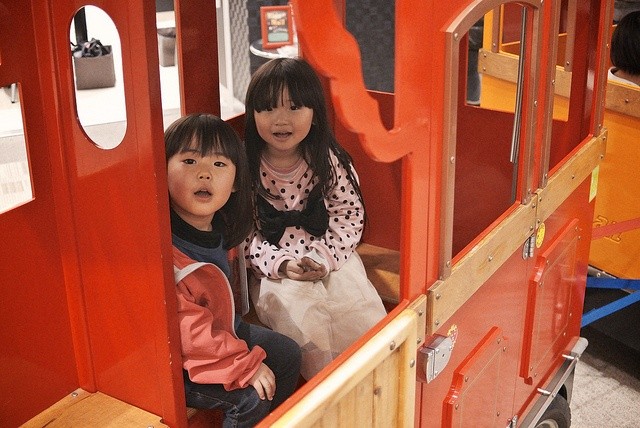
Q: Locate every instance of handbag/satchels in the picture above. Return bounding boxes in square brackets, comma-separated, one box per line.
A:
[81, 38, 108, 57]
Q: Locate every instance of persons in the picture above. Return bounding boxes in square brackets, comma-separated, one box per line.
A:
[608, 10, 640, 89]
[165, 113, 303, 427]
[240, 58, 387, 384]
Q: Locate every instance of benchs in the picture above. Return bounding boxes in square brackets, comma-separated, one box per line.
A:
[175, 243, 401, 418]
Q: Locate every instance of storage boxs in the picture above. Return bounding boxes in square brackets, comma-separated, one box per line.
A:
[157, 27, 177, 66]
[73, 45, 116, 90]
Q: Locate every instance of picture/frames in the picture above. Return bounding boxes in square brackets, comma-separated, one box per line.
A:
[259, 5, 294, 49]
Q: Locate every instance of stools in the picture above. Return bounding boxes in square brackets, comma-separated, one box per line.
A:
[18, 386, 172, 428]
